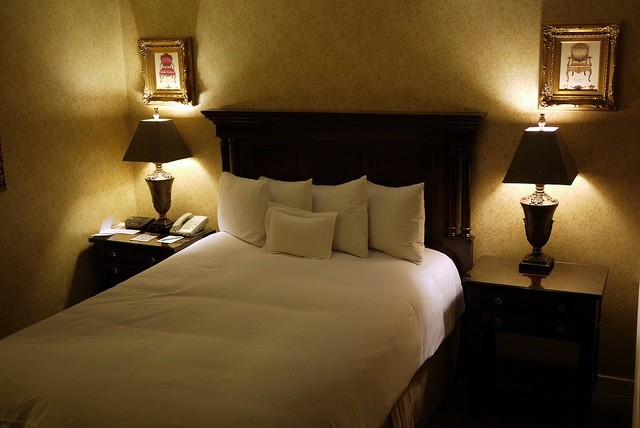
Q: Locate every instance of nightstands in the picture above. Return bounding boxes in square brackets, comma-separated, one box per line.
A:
[463, 254, 610, 403]
[90, 220, 215, 292]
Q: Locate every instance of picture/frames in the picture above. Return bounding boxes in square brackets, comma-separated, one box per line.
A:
[138, 38, 197, 106]
[538, 24, 622, 112]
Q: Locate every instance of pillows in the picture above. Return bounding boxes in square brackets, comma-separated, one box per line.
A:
[256, 176, 312, 213]
[313, 174, 367, 258]
[218, 170, 269, 248]
[367, 182, 426, 265]
[262, 202, 338, 262]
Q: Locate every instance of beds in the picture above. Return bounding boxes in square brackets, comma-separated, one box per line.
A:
[1, 109, 488, 427]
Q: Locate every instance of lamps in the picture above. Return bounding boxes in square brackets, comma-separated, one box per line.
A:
[501, 113, 578, 276]
[122, 109, 193, 233]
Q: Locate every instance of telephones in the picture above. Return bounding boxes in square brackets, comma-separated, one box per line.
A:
[170, 212, 209, 237]
